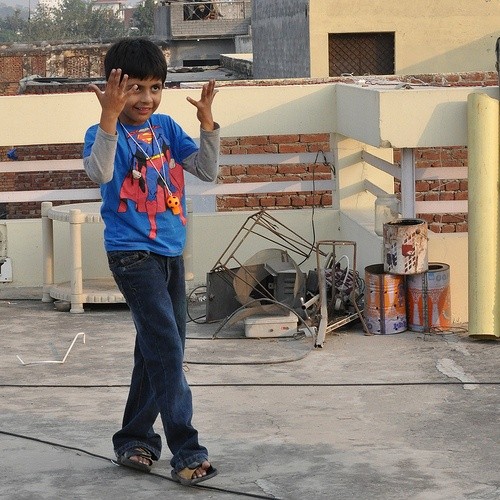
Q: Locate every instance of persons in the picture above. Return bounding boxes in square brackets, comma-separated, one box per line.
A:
[82, 37, 224, 488]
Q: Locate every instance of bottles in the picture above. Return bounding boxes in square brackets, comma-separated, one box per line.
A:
[375, 194, 400, 236]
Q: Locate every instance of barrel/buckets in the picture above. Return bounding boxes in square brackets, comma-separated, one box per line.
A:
[363, 264, 407, 335]
[383, 217, 428, 275]
[407, 262, 451, 333]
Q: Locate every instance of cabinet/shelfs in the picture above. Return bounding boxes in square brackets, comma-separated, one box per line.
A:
[41, 197, 194, 314]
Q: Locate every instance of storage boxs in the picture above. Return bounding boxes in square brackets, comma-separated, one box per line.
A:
[244, 311, 298, 338]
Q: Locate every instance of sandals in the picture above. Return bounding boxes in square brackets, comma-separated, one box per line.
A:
[117, 447, 153, 472]
[171, 462, 218, 486]
